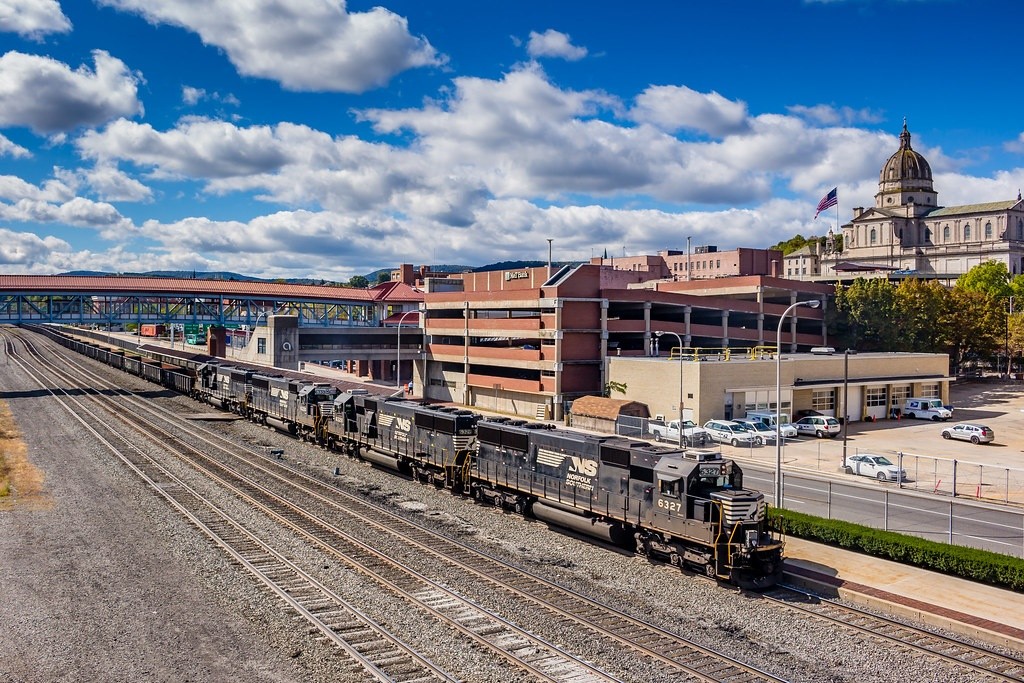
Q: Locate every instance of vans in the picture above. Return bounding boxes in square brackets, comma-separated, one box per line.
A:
[902, 397, 953, 420]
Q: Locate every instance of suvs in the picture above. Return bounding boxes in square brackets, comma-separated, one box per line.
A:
[729, 418, 774, 446]
[794, 414, 841, 439]
[704, 419, 766, 447]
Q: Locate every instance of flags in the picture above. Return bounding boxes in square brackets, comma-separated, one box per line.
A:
[814, 187, 838, 219]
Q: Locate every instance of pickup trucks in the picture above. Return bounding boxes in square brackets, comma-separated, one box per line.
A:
[648, 418, 707, 446]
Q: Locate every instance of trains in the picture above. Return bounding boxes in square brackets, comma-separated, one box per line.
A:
[18, 322, 785, 593]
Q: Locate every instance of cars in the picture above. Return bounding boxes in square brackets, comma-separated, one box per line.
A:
[769, 424, 798, 438]
[839, 453, 907, 481]
[940, 422, 994, 445]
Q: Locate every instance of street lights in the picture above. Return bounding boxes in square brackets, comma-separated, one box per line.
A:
[397, 307, 427, 388]
[654, 329, 683, 449]
[776, 300, 821, 508]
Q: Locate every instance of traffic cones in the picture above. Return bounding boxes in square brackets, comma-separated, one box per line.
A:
[898, 412, 900, 421]
[872, 414, 876, 424]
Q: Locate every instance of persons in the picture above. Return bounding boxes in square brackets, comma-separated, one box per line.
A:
[408, 381, 413, 395]
